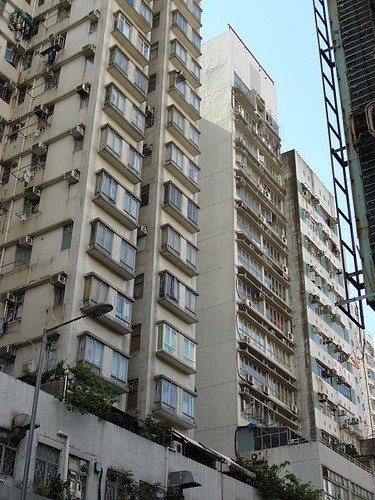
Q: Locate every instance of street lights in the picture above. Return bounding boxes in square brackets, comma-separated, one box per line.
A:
[20, 301, 114, 500]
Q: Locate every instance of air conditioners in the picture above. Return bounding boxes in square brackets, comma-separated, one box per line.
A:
[69, 125, 84, 140]
[64, 170, 80, 184]
[0, 344, 16, 360]
[88, 9, 100, 21]
[176, 70, 187, 82]
[170, 440, 182, 455]
[21, 360, 38, 376]
[142, 142, 152, 152]
[34, 104, 49, 118]
[40, 67, 54, 80]
[50, 274, 67, 288]
[0, 291, 15, 305]
[76, 84, 90, 96]
[31, 141, 48, 156]
[137, 226, 147, 238]
[81, 43, 96, 59]
[145, 105, 153, 116]
[18, 236, 33, 249]
[235, 101, 361, 463]
[23, 186, 41, 202]
